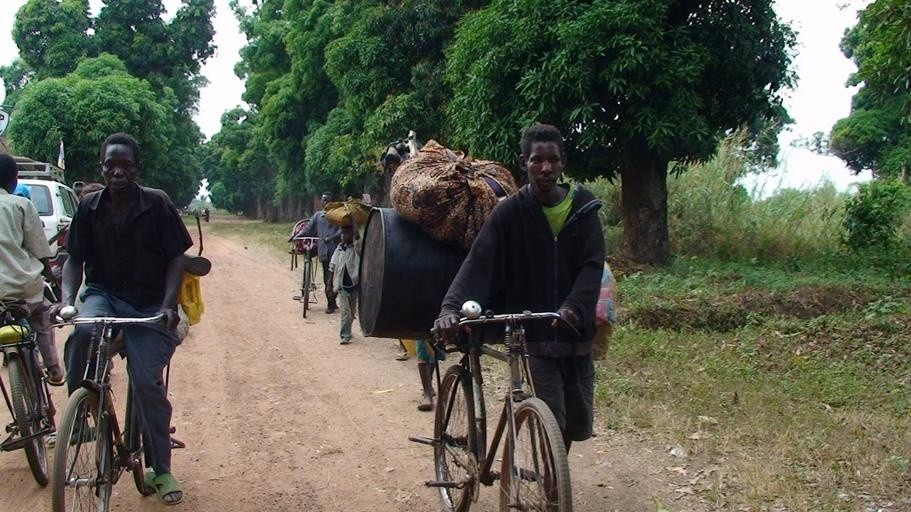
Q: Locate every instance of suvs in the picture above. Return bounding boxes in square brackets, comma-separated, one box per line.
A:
[15, 159, 83, 269]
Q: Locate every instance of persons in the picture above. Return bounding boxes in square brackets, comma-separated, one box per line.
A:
[0, 154, 64, 387]
[205, 207, 210, 223]
[393, 338, 409, 360]
[436, 124, 607, 512]
[78, 182, 107, 203]
[325, 209, 366, 346]
[299, 191, 342, 314]
[44, 131, 195, 507]
[415, 338, 447, 409]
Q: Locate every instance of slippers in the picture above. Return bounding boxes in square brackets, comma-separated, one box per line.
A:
[396, 353, 408, 361]
[418, 401, 433, 410]
[144, 470, 184, 506]
[46, 367, 66, 387]
[45, 428, 97, 449]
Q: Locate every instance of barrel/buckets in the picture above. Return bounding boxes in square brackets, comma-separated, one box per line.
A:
[358, 206, 506, 344]
[177, 273, 204, 328]
[291, 218, 317, 251]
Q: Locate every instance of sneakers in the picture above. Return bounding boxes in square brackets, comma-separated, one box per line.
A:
[326, 308, 334, 314]
[340, 340, 349, 344]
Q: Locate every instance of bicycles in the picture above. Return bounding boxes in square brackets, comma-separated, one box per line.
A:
[43, 304, 186, 512]
[287, 217, 326, 320]
[0, 275, 66, 489]
[408, 298, 584, 512]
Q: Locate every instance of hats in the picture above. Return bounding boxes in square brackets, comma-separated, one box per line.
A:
[13, 184, 30, 199]
